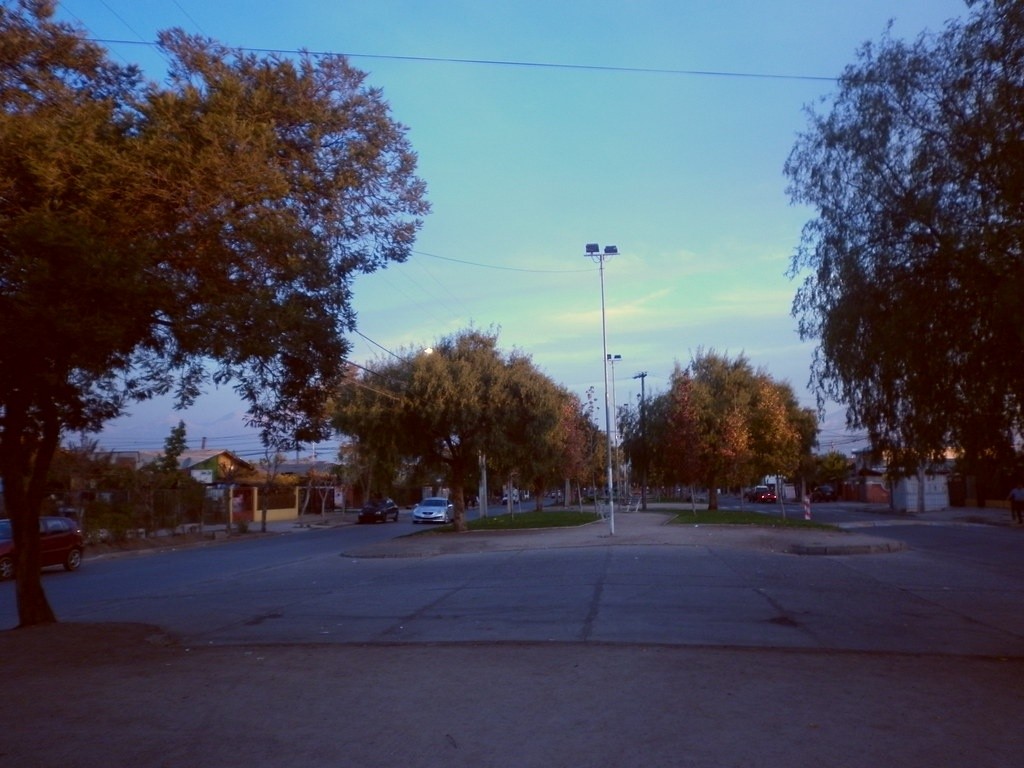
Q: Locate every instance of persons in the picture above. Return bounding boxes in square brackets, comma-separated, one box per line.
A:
[471, 494, 476, 510]
[1009, 481, 1024, 523]
[465, 495, 471, 510]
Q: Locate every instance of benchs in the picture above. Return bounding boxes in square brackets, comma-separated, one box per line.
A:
[618, 496, 642, 512]
[598, 502, 608, 520]
[179, 523, 200, 535]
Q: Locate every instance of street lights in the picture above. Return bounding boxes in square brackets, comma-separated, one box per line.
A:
[583, 244, 621, 536]
[606, 353, 623, 514]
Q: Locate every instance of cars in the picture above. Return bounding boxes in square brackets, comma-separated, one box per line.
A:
[357, 497, 400, 525]
[0, 516, 87, 582]
[551, 490, 561, 499]
[810, 486, 839, 504]
[743, 486, 777, 505]
[411, 496, 454, 524]
[503, 493, 517, 505]
